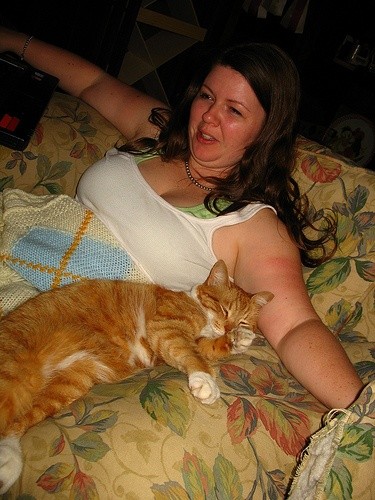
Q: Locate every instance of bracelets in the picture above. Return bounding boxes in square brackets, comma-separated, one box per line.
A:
[20, 34, 34, 61]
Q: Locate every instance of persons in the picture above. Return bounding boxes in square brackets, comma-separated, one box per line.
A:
[0, 28, 365, 412]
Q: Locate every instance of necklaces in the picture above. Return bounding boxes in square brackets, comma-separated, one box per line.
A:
[183, 158, 219, 192]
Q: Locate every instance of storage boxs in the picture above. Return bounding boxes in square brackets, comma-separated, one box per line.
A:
[0, 51, 60, 153]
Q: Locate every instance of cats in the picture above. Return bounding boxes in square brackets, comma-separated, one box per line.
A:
[0, 260, 275, 495]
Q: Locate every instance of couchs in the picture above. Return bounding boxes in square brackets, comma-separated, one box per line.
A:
[0, 90, 375, 500]
